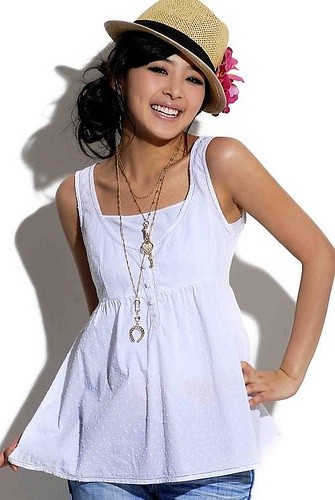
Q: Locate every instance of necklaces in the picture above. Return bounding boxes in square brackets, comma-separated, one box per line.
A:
[114, 132, 186, 342]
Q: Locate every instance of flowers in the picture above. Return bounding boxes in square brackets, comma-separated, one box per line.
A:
[213, 47, 246, 116]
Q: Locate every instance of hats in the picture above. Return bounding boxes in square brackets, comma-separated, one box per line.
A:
[104, 0, 245, 116]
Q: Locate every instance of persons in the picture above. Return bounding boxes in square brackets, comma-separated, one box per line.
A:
[0, 0, 335, 500]
